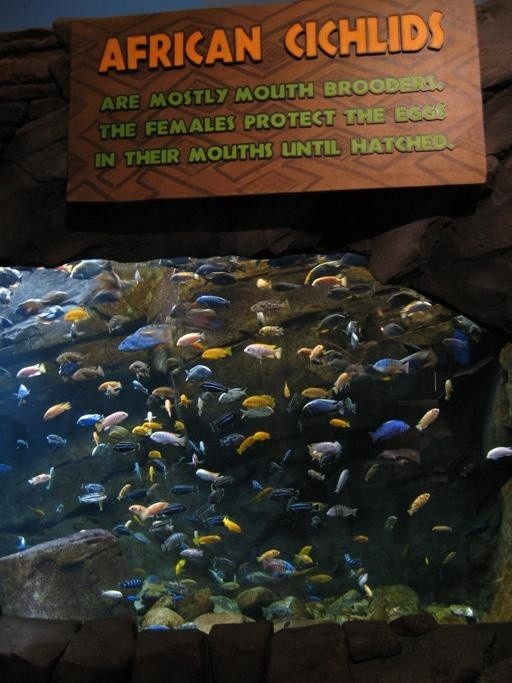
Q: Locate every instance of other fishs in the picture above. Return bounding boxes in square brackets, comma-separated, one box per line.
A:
[0, 252, 485, 632]
